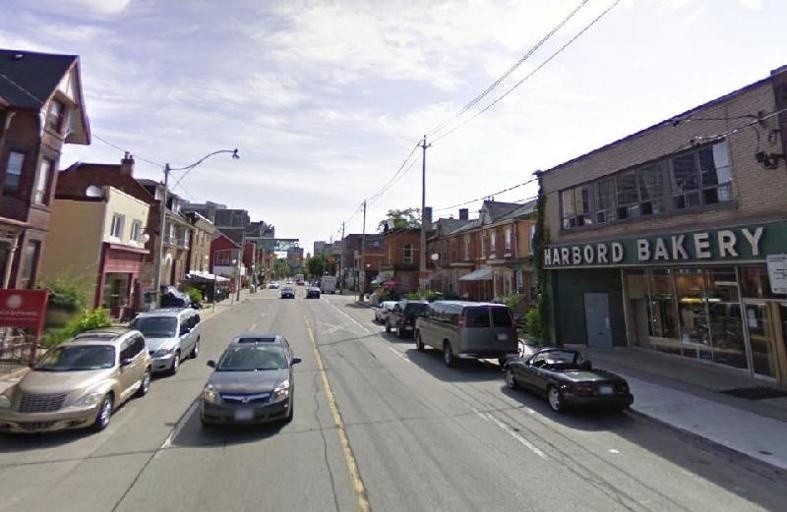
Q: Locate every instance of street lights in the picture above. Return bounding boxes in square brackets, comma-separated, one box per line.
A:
[151, 148, 240, 306]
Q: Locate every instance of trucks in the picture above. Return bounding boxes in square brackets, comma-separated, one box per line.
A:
[320, 276, 337, 294]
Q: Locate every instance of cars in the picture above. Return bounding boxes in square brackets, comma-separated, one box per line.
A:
[374, 300, 398, 324]
[281, 288, 296, 298]
[269, 281, 279, 289]
[502, 347, 633, 414]
[199, 333, 302, 427]
[131, 307, 201, 375]
[0, 324, 154, 434]
[306, 281, 320, 299]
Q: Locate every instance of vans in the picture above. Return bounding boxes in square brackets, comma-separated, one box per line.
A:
[386, 299, 518, 368]
